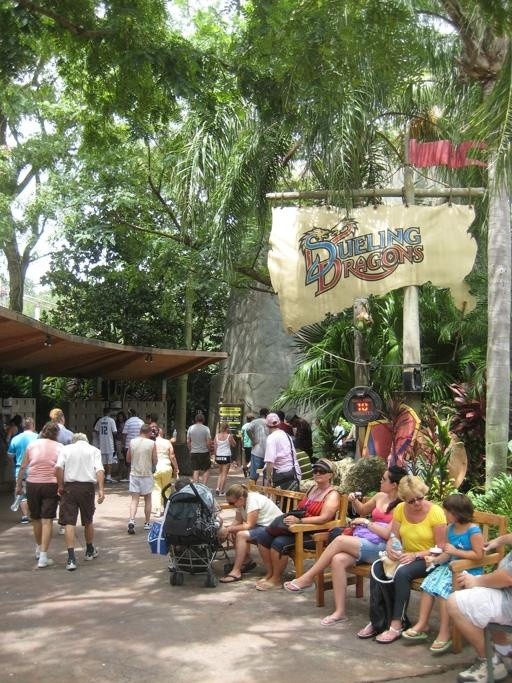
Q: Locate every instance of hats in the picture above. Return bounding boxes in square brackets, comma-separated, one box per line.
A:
[285, 411, 296, 423]
[265, 413, 280, 427]
[312, 462, 333, 473]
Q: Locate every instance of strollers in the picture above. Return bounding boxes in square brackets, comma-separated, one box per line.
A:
[161, 479, 227, 590]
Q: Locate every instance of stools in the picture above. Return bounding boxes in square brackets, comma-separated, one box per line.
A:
[484, 623, 511, 683]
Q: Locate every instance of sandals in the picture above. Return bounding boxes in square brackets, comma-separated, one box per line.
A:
[356, 621, 404, 644]
[241, 559, 256, 572]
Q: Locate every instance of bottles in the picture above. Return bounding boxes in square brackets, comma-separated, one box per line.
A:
[10, 494, 23, 512]
[390, 533, 402, 554]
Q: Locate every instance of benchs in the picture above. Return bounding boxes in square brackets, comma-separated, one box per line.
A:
[312, 496, 506, 654]
[220, 479, 349, 577]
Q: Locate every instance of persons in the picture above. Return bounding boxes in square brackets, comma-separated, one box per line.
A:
[1, 408, 236, 571]
[283, 466, 512, 682]
[217, 408, 341, 591]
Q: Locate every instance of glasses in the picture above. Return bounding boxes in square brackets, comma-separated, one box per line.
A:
[405, 494, 423, 504]
[314, 468, 333, 475]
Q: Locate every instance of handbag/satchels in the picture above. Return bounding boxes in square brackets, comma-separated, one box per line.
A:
[273, 479, 300, 505]
[148, 522, 168, 555]
[382, 555, 405, 579]
[265, 510, 305, 536]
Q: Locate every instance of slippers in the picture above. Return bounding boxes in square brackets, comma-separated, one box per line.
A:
[219, 574, 242, 583]
[402, 628, 428, 639]
[430, 640, 452, 652]
[284, 578, 315, 593]
[255, 578, 283, 591]
[321, 614, 348, 625]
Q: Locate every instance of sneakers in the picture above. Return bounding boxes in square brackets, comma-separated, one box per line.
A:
[144, 522, 152, 530]
[242, 465, 248, 478]
[35, 545, 99, 571]
[457, 647, 512, 683]
[216, 488, 226, 497]
[105, 477, 118, 483]
[21, 517, 29, 523]
[128, 522, 136, 533]
[120, 478, 130, 482]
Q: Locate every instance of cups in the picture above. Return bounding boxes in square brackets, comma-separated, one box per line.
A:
[430, 548, 442, 558]
